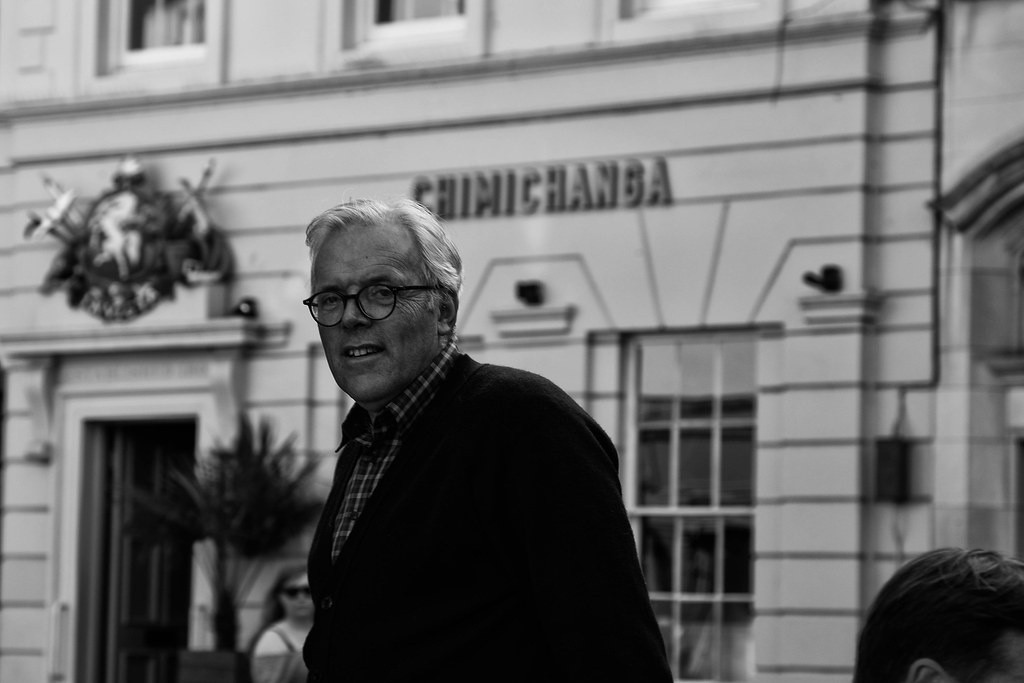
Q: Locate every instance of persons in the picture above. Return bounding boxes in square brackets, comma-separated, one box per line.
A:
[853, 547, 1024, 683]
[303, 201, 674, 682]
[249, 566, 313, 683]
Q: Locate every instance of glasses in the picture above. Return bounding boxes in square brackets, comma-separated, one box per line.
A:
[303, 282, 441, 327]
[281, 585, 311, 599]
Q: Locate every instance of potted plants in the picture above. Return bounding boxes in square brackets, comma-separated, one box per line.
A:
[123, 410, 329, 683]
